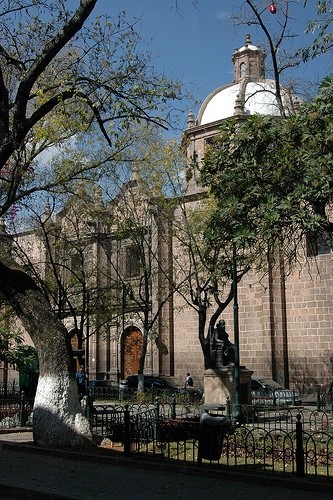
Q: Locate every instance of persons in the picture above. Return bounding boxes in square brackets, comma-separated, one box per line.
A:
[76, 367, 87, 395]
[185, 372, 193, 389]
[213, 320, 234, 364]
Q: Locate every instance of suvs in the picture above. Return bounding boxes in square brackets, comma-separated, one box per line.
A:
[126, 372, 200, 402]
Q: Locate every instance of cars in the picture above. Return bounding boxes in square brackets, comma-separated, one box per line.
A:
[251, 376, 302, 405]
[88, 379, 120, 396]
